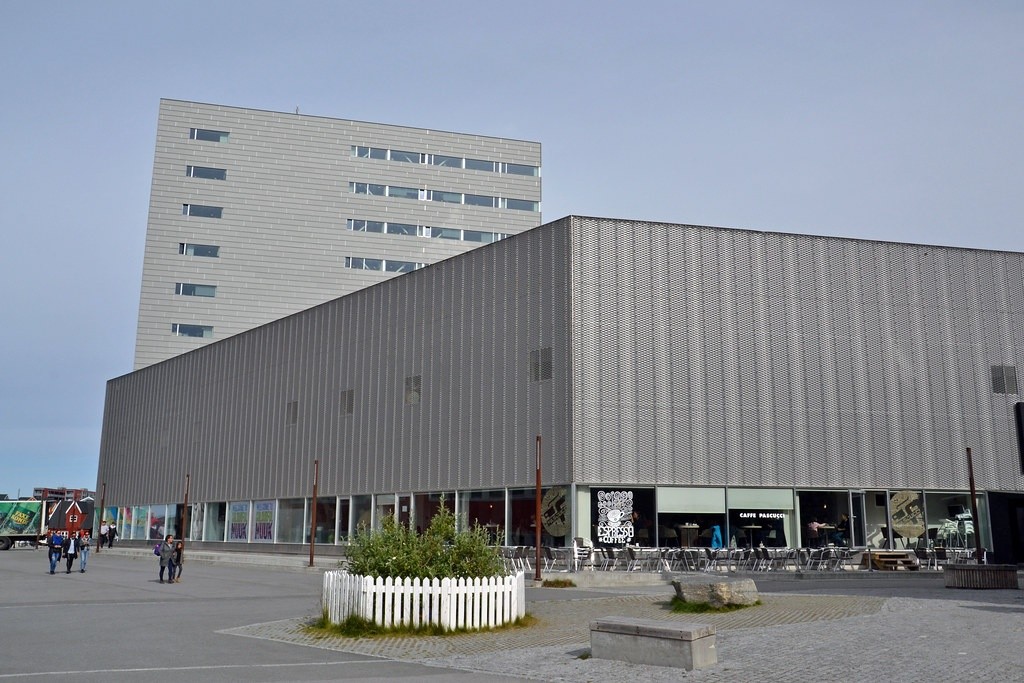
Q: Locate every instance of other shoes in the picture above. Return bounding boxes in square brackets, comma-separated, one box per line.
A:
[50, 571, 55, 574]
[172, 579, 176, 583]
[176, 577, 180, 583]
[160, 580, 164, 584]
[67, 570, 71, 574]
[81, 569, 85, 573]
[168, 579, 173, 583]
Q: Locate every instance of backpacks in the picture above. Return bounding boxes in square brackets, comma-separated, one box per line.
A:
[153, 544, 161, 556]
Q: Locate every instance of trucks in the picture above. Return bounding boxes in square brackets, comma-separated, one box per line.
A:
[0, 501, 47, 550]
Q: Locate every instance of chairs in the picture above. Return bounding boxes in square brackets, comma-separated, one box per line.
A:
[881, 527, 906, 549]
[913, 505, 987, 572]
[492, 525, 856, 574]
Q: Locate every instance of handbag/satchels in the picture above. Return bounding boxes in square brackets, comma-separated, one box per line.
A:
[81, 545, 84, 550]
[117, 536, 118, 541]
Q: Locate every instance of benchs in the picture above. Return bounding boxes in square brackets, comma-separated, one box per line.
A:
[590, 615, 718, 669]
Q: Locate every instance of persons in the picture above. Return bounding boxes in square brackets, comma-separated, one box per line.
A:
[47, 530, 63, 574]
[517, 510, 538, 546]
[831, 513, 850, 546]
[78, 531, 93, 574]
[64, 532, 80, 574]
[97, 521, 109, 549]
[631, 511, 646, 547]
[762, 518, 783, 547]
[172, 541, 185, 583]
[158, 535, 176, 583]
[806, 517, 827, 548]
[107, 521, 119, 549]
